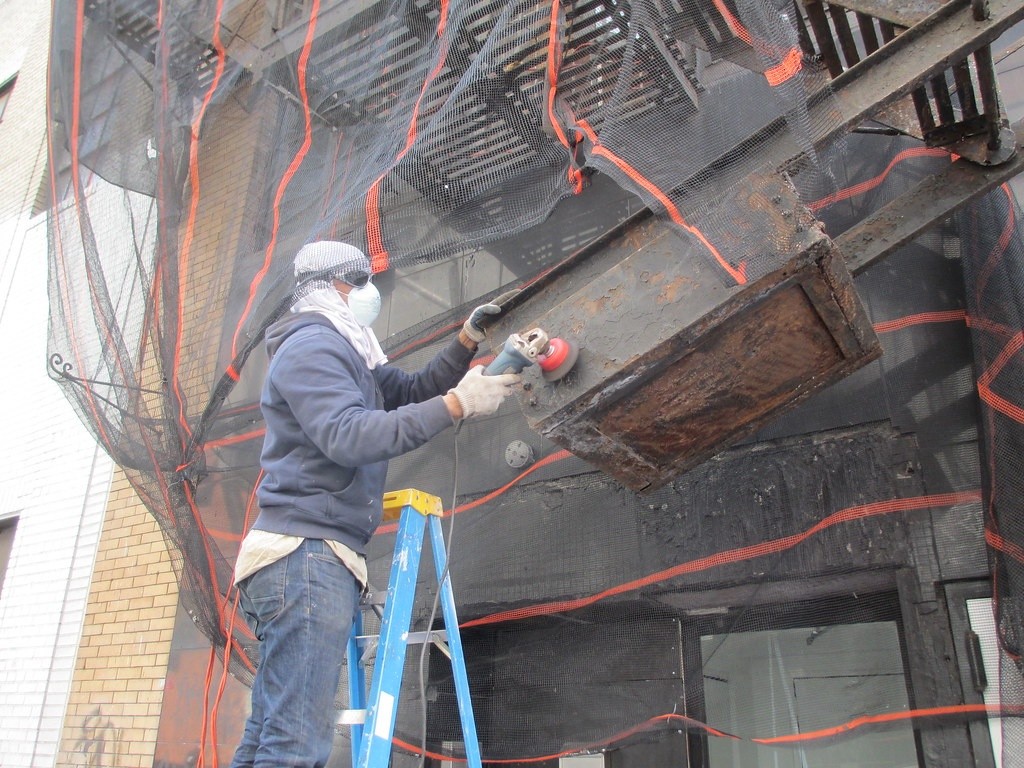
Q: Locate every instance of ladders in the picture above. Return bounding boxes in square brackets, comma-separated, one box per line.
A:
[333, 486, 482, 767]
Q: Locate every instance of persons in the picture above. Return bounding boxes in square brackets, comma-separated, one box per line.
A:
[223, 241, 524, 768]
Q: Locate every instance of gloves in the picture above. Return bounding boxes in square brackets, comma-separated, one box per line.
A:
[464, 288, 522, 343]
[447, 364, 523, 421]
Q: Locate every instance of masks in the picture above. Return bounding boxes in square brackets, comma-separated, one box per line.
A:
[330, 277, 381, 328]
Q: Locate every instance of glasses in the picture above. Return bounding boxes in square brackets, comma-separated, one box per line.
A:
[332, 271, 370, 289]
